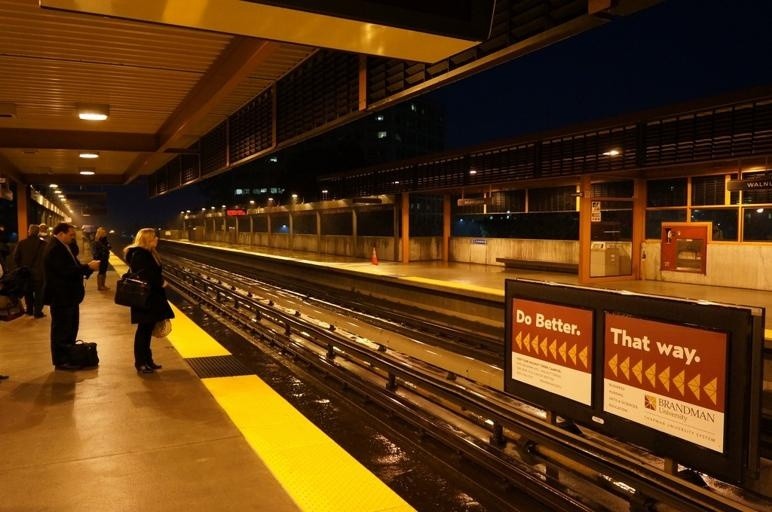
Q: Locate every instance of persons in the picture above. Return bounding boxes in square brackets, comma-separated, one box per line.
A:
[0, 223, 48, 318]
[41, 223, 101, 370]
[92, 226, 112, 290]
[122, 227, 174, 373]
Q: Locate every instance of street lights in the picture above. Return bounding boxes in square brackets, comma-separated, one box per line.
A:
[152, 318, 173, 338]
[115, 273, 152, 308]
[0, 293, 26, 322]
[6, 266, 38, 298]
[70, 339, 99, 368]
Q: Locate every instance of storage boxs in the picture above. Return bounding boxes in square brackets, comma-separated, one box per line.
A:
[495, 257, 579, 278]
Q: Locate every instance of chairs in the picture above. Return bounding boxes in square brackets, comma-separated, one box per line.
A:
[25, 310, 47, 319]
[56, 363, 81, 371]
[137, 362, 163, 373]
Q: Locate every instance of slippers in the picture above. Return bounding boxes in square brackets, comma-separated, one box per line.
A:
[91, 240, 106, 260]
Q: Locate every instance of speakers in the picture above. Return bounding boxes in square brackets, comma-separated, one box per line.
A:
[97, 273, 111, 291]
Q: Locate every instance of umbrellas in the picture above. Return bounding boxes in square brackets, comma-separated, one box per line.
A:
[1, 374, 9, 381]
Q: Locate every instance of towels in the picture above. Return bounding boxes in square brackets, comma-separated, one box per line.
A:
[590, 240, 631, 277]
